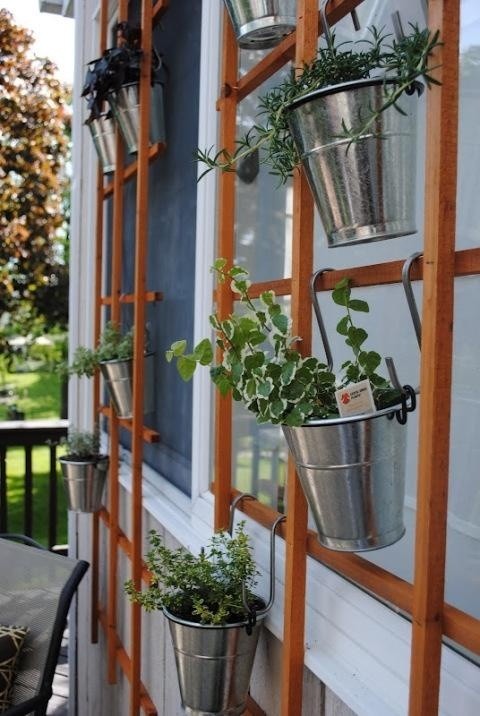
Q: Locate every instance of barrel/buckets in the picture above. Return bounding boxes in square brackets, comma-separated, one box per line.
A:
[282, 70, 423, 246]
[163, 586, 270, 716]
[281, 385, 420, 553]
[104, 85, 167, 153]
[59, 452, 108, 515]
[85, 113, 117, 171]
[224, 0, 297, 48]
[101, 352, 156, 422]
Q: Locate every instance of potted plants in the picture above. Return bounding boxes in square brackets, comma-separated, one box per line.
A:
[164, 256, 412, 551]
[188, 23, 445, 247]
[123, 520, 269, 716]
[53, 319, 156, 419]
[81, 20, 172, 174]
[43, 431, 126, 514]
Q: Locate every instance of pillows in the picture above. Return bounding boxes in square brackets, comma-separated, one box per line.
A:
[0, 624, 31, 716]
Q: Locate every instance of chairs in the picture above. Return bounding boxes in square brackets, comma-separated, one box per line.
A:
[0, 534, 90, 716]
